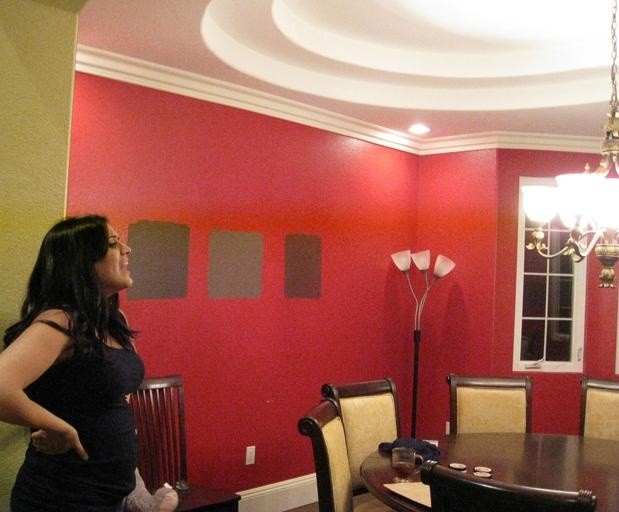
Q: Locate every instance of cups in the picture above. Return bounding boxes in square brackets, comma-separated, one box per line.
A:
[448, 461, 468, 473]
[473, 466, 493, 473]
[389, 445, 426, 483]
[473, 471, 492, 480]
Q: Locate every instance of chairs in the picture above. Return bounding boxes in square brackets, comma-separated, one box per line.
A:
[320, 377, 401, 511]
[130, 374, 241, 511]
[445, 372, 533, 434]
[297, 398, 353, 512]
[578, 376, 619, 442]
[420, 460, 596, 511]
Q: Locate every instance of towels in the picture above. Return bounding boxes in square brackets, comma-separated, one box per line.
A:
[378, 436, 441, 460]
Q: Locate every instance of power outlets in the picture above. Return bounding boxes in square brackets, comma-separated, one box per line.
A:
[245, 446, 256, 465]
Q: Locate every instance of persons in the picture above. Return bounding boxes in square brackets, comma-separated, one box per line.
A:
[0, 215, 145, 511]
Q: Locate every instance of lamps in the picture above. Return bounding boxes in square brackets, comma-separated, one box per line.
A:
[519, 1, 619, 288]
[390, 249, 457, 438]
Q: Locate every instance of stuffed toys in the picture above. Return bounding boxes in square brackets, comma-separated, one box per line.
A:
[117, 464, 179, 512]
